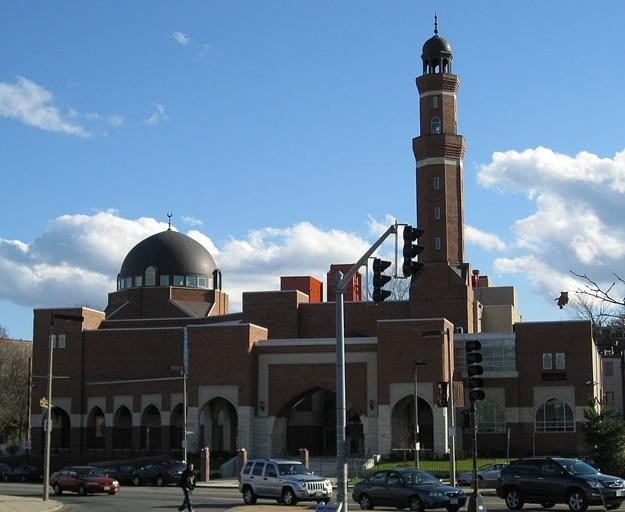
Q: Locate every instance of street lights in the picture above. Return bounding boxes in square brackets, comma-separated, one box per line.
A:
[413, 359, 429, 468]
[422, 328, 456, 486]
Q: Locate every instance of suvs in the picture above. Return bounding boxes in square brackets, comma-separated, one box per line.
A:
[239, 457, 333, 506]
[496, 456, 625, 512]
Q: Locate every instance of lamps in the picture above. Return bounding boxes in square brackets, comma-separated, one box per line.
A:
[368, 399, 375, 409]
[260, 398, 265, 412]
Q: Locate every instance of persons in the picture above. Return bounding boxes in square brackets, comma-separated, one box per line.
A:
[178, 461, 197, 512]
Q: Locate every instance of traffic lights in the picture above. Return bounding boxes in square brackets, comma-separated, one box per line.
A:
[372, 258, 392, 303]
[465, 340, 485, 403]
[402, 225, 425, 277]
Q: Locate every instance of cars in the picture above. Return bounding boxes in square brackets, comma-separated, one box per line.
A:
[0, 460, 187, 497]
[457, 462, 509, 486]
[352, 468, 467, 512]
